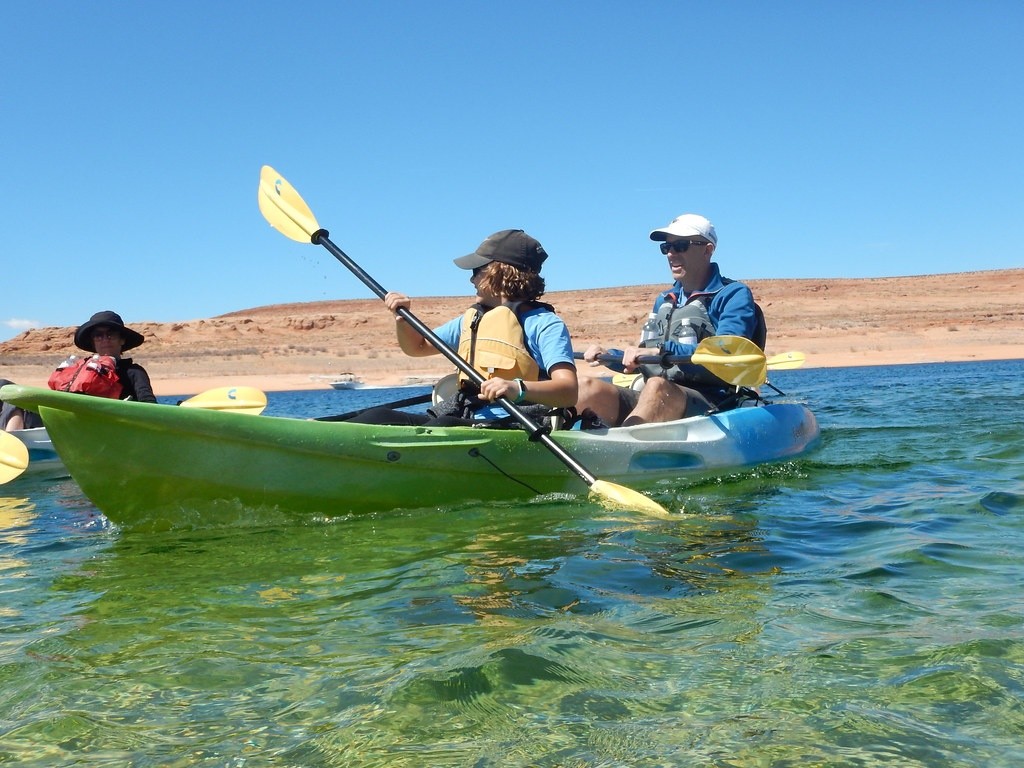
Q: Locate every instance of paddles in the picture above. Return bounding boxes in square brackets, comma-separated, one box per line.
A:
[570, 334, 770, 387]
[254, 163, 674, 519]
[612, 351, 807, 388]
[174, 383, 269, 417]
[0, 429, 30, 486]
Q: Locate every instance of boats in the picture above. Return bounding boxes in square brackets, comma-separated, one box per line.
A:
[0, 382, 821, 531]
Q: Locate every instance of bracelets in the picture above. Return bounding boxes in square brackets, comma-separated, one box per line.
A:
[512, 378, 526, 404]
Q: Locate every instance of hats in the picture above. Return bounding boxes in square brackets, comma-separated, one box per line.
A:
[453, 228, 549, 273]
[73, 310, 145, 352]
[648, 213, 718, 248]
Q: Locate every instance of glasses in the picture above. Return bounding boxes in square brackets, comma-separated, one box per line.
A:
[660, 239, 708, 255]
[471, 268, 488, 277]
[91, 330, 122, 342]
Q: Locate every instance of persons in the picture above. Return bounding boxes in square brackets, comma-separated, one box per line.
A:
[564, 214, 767, 428]
[0, 379, 25, 431]
[74, 311, 158, 404]
[343, 229, 578, 427]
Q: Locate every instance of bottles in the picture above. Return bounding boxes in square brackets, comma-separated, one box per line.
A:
[679, 318, 697, 345]
[640, 312, 660, 343]
